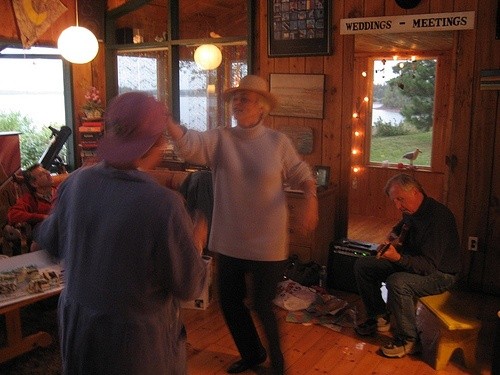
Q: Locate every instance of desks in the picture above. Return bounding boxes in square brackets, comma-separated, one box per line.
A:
[0, 250, 64, 364]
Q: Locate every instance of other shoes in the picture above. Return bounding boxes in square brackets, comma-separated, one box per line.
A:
[227, 350, 266, 374]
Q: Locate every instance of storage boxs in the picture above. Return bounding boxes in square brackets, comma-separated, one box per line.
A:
[178, 257, 216, 312]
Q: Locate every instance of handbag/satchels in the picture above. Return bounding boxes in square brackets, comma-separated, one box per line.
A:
[272, 254, 321, 310]
[178, 255, 218, 310]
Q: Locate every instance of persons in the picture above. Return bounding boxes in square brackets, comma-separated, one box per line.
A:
[161, 75, 319, 375]
[39, 92, 208, 375]
[8, 163, 56, 230]
[353, 173, 460, 357]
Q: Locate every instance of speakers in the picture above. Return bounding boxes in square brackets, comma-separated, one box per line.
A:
[325, 239, 379, 296]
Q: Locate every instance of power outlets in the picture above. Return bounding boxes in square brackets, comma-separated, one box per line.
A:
[468, 236, 478, 253]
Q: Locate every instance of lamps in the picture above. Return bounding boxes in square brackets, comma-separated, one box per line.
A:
[57, 0, 99, 65]
[193, 43, 223, 70]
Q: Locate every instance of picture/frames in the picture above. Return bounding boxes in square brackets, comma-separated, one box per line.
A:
[314, 165, 331, 189]
[268, 73, 325, 119]
[267, 0, 333, 58]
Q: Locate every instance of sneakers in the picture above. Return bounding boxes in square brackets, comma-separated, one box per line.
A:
[355, 316, 390, 336]
[381, 337, 419, 357]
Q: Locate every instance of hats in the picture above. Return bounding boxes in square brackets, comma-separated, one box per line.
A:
[96, 91, 168, 164]
[222, 74, 276, 111]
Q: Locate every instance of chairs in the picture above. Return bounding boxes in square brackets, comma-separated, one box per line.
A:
[5, 171, 67, 254]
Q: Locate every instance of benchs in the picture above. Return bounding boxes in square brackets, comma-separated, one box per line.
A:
[420, 291, 484, 372]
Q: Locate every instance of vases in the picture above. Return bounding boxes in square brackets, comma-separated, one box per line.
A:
[84, 108, 103, 117]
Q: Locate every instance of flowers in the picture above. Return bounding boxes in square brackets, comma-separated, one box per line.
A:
[85, 87, 104, 110]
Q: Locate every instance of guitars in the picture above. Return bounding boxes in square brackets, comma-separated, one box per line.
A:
[375, 222, 411, 260]
